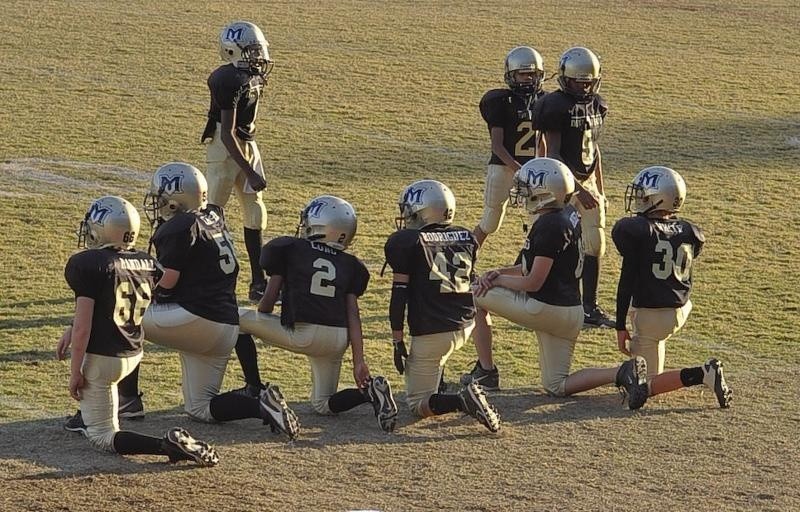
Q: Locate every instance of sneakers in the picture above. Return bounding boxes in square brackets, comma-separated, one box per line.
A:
[258, 385, 299, 441]
[438, 378, 448, 393]
[616, 356, 648, 410]
[64, 409, 89, 438]
[366, 375, 398, 432]
[231, 382, 270, 400]
[460, 360, 500, 392]
[457, 382, 501, 433]
[119, 391, 145, 419]
[583, 306, 616, 330]
[701, 358, 732, 408]
[160, 425, 220, 467]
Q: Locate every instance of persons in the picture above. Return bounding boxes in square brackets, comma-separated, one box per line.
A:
[380, 177, 502, 433]
[459, 157, 649, 412]
[117, 161, 302, 442]
[611, 165, 733, 411]
[234, 194, 398, 433]
[472, 45, 551, 248]
[53, 193, 219, 468]
[198, 20, 283, 305]
[530, 45, 616, 331]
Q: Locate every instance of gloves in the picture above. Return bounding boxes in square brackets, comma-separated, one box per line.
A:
[391, 338, 409, 374]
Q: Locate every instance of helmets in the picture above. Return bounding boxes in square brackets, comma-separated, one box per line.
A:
[503, 46, 545, 84]
[557, 46, 603, 93]
[83, 195, 141, 251]
[151, 161, 210, 222]
[514, 156, 576, 216]
[397, 180, 458, 231]
[301, 194, 357, 251]
[628, 165, 687, 215]
[219, 22, 270, 68]
